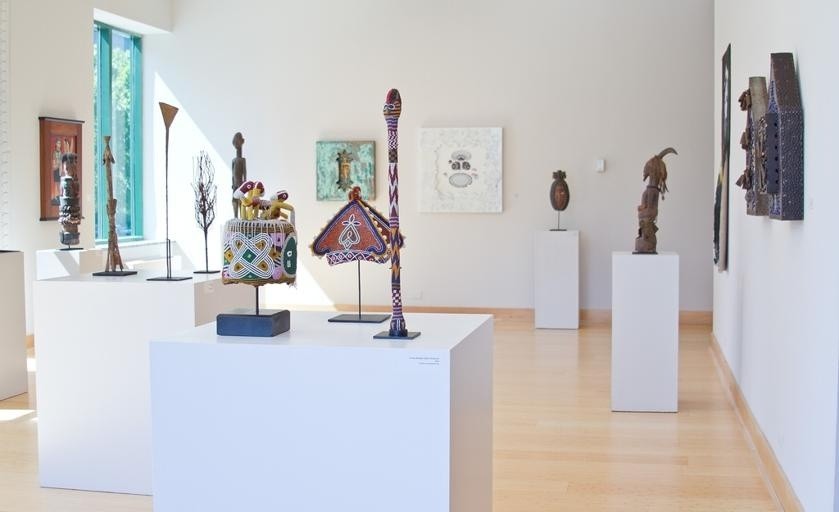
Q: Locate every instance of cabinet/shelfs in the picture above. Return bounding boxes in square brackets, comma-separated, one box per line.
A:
[32, 273, 251, 497]
[148, 310, 495, 512]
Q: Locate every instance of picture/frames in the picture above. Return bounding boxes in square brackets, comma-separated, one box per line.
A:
[38, 115, 86, 222]
